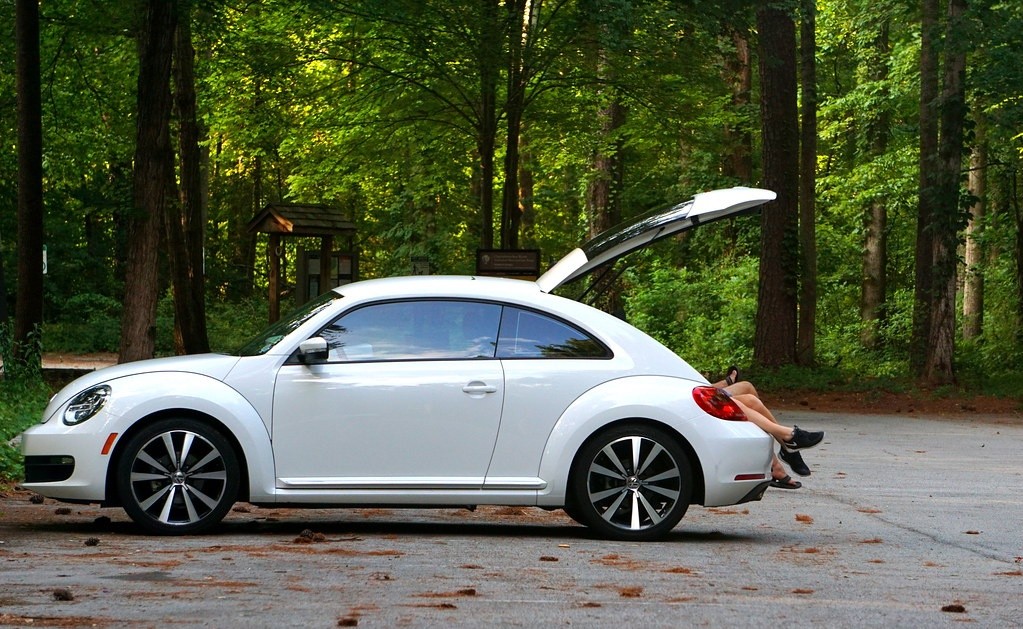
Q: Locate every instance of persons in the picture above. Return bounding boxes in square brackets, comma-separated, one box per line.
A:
[710, 368, 824, 490]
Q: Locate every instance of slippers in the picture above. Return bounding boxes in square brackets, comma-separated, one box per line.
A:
[726, 365, 739, 385]
[769, 474, 802, 489]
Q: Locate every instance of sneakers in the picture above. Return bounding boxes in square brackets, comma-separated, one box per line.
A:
[777, 443, 811, 476]
[782, 424, 826, 453]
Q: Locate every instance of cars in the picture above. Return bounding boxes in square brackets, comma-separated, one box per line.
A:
[18, 185, 780, 540]
